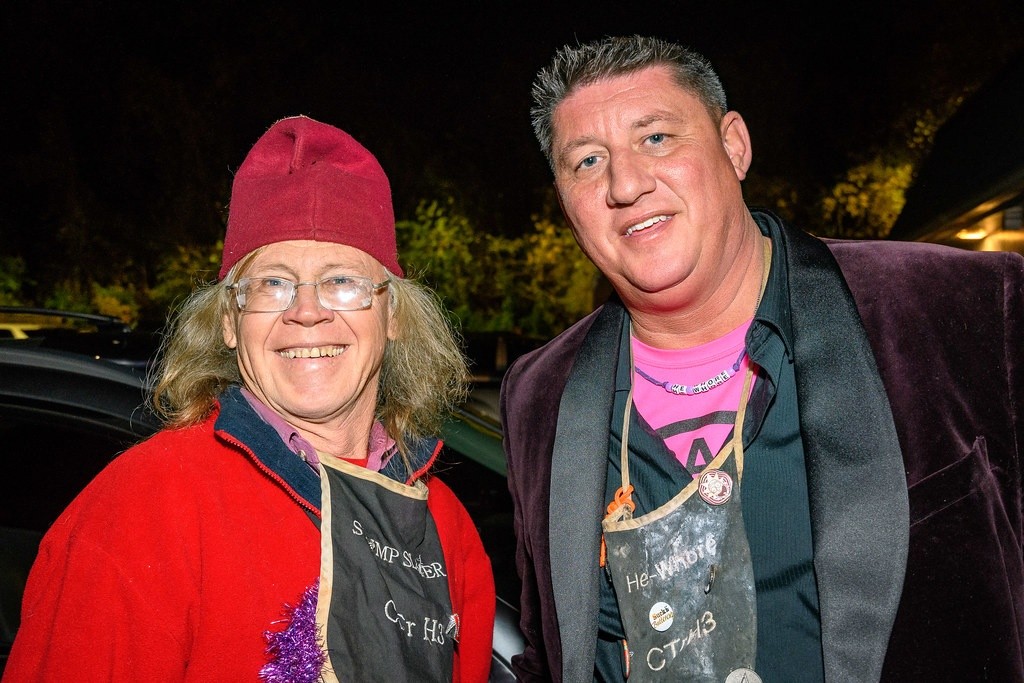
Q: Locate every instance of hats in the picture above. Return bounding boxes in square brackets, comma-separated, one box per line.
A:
[218, 116, 404, 280]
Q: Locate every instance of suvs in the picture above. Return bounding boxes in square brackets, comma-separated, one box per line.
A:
[0, 305, 541, 683]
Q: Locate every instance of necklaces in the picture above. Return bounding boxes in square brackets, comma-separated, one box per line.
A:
[636, 348, 748, 395]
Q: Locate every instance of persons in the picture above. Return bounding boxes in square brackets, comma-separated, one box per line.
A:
[2, 114, 496, 683]
[497, 36, 1023, 682]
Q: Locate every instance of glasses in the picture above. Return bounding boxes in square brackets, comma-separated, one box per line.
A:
[230, 275, 392, 313]
